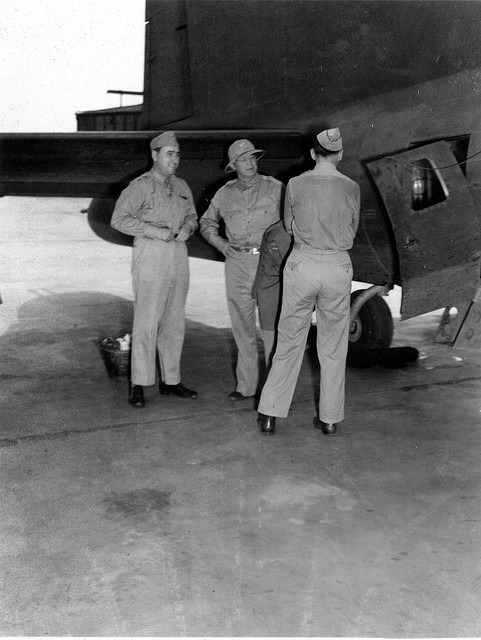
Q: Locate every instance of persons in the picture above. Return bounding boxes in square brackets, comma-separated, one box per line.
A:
[198, 139, 285, 401]
[257, 128, 362, 434]
[109, 128, 199, 408]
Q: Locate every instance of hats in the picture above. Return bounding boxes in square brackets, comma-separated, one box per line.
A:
[225, 140, 267, 174]
[151, 131, 180, 152]
[318, 127, 344, 153]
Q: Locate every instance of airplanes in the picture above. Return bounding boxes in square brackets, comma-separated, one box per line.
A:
[0, 0, 480, 367]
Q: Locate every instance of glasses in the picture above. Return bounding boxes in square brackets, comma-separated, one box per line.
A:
[237, 157, 259, 164]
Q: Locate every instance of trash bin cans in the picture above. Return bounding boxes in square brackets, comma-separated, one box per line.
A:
[98, 334, 132, 380]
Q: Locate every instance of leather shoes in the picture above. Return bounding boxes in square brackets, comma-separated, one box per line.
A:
[159, 381, 199, 400]
[314, 417, 339, 437]
[227, 391, 254, 402]
[258, 414, 278, 436]
[129, 382, 146, 408]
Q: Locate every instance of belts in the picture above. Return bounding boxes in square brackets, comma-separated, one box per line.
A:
[231, 246, 261, 255]
